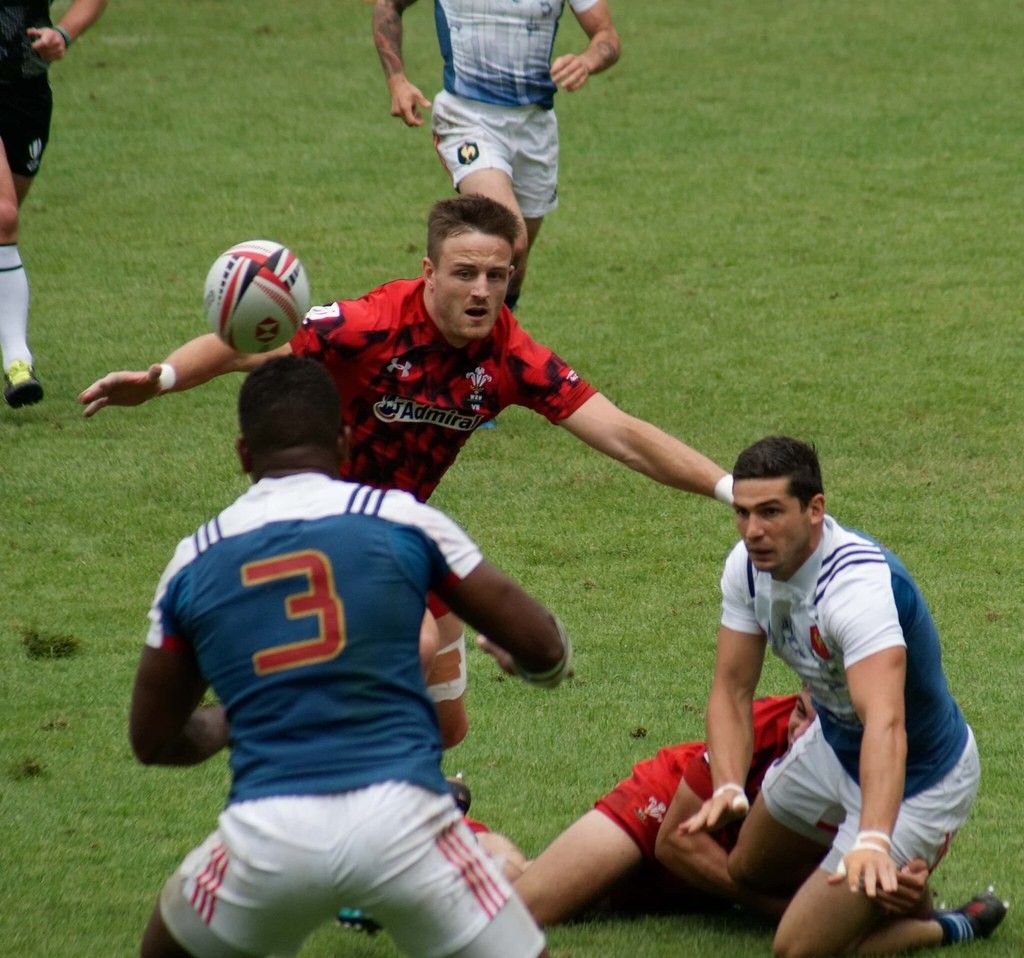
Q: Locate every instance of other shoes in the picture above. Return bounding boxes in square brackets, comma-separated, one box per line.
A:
[445, 777, 472, 816]
[335, 906, 381, 938]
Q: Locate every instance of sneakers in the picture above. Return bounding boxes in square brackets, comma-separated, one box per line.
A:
[954, 891, 1006, 941]
[3, 361, 45, 410]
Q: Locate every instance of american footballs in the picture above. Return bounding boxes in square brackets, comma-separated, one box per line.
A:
[201, 238, 312, 356]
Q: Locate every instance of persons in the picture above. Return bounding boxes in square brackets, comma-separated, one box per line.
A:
[76, 191, 739, 938]
[128, 354, 571, 958]
[460, 676, 925, 931]
[0, 0, 107, 408]
[371, 0, 621, 314]
[671, 431, 1010, 958]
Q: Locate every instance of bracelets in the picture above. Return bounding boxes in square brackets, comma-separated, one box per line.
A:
[51, 22, 71, 49]
